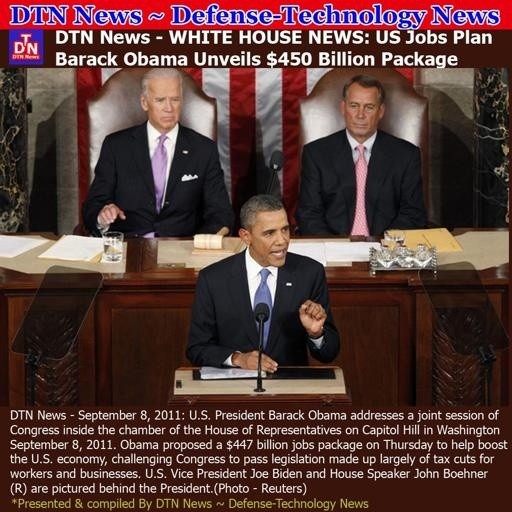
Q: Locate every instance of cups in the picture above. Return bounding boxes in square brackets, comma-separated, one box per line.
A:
[376, 231, 433, 270]
[102, 231, 124, 264]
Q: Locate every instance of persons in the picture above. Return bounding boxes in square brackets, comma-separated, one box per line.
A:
[80, 69, 235, 241]
[182, 194, 341, 374]
[290, 72, 425, 236]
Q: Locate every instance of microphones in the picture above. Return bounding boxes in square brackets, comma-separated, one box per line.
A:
[253, 299, 272, 394]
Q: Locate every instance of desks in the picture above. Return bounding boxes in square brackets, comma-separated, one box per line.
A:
[4, 234, 509, 405]
[261, 405, 264, 407]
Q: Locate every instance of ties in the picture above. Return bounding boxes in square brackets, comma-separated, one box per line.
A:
[142, 135, 168, 238]
[352, 145, 371, 237]
[254, 268, 273, 350]
